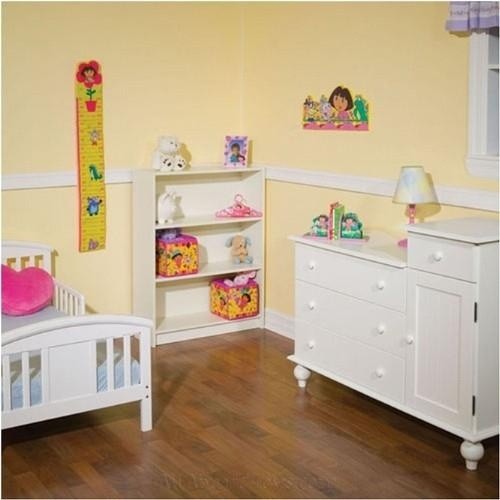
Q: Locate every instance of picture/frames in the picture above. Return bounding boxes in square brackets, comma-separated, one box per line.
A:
[223, 135, 249, 168]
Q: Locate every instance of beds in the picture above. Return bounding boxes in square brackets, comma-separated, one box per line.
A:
[0, 241, 155, 432]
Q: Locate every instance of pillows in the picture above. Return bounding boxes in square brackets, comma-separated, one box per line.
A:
[0, 265, 54, 316]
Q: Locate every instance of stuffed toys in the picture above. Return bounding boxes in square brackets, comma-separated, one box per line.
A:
[157, 187, 177, 224]
[226, 235, 253, 264]
[153, 135, 187, 173]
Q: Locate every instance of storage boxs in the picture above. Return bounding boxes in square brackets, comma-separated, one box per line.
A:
[210, 278, 259, 320]
[156, 235, 199, 278]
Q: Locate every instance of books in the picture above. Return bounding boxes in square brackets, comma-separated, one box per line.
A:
[328, 201, 339, 240]
[333, 205, 345, 238]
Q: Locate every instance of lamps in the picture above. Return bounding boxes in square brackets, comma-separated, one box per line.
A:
[392, 167, 434, 247]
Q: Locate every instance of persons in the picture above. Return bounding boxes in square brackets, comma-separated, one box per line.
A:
[230, 144, 240, 162]
[341, 212, 363, 239]
[310, 213, 328, 236]
[218, 194, 263, 218]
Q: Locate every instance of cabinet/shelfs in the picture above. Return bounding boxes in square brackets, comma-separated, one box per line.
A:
[132, 164, 265, 348]
[286, 217, 499, 471]
